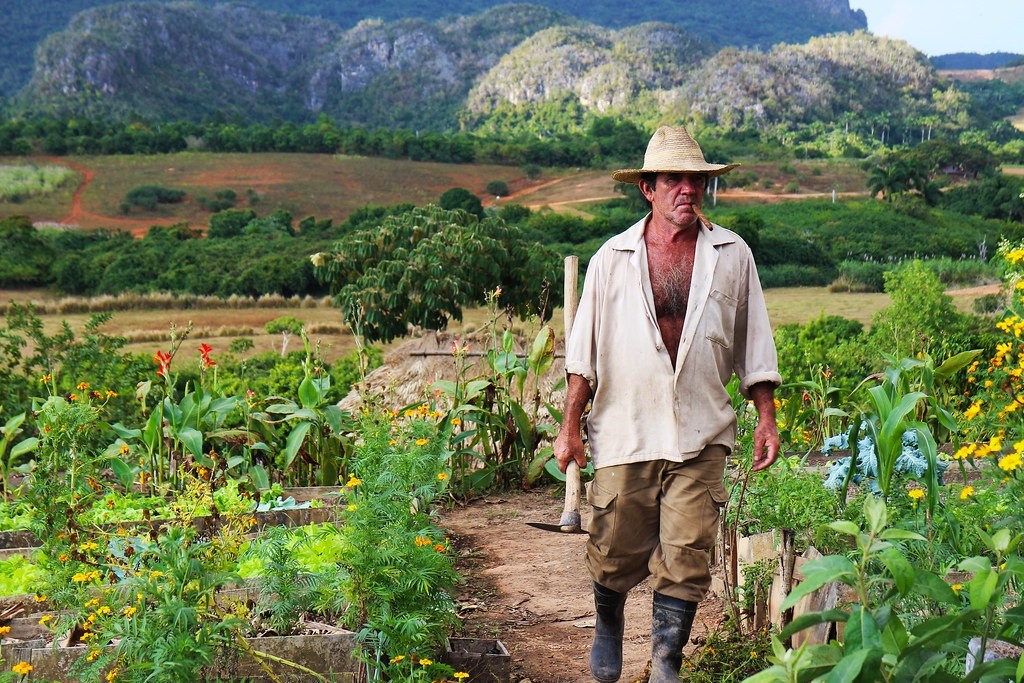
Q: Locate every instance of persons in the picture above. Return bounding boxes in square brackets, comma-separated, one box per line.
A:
[553, 126, 783, 683]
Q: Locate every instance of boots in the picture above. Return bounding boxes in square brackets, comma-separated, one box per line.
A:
[589, 586, 624, 682]
[647, 590, 697, 682]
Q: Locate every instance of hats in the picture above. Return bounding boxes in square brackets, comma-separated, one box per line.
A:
[612, 125, 741, 182]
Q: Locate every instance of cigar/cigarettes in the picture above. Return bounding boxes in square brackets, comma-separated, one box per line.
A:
[691, 203, 714, 232]
